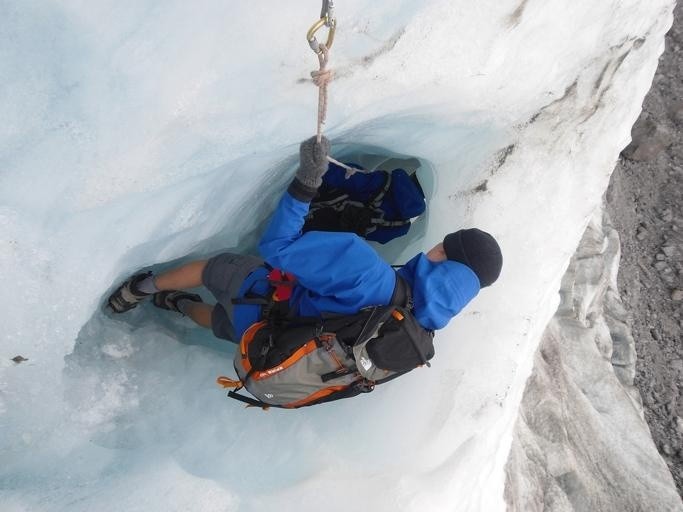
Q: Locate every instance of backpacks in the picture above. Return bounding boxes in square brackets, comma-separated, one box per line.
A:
[232, 275, 436, 410]
[304, 173, 410, 237]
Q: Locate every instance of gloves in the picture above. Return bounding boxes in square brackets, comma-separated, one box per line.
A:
[297, 135, 331, 188]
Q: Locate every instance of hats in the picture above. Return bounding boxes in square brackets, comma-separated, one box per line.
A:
[444, 228, 503, 287]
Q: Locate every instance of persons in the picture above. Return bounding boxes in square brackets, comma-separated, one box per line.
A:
[307, 157, 434, 243]
[99, 131, 502, 353]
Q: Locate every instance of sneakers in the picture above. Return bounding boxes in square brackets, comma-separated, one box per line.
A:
[152, 289, 202, 313]
[108, 272, 154, 313]
